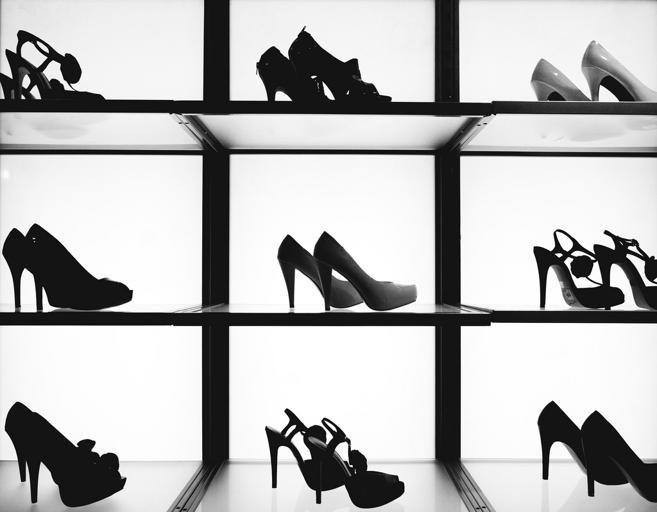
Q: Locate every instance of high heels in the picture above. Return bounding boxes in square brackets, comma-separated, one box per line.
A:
[277, 235, 363, 308]
[314, 231, 417, 310]
[1, 30, 103, 100]
[582, 410, 656, 503]
[257, 46, 335, 101]
[538, 401, 629, 484]
[582, 40, 656, 101]
[534, 229, 625, 309]
[288, 25, 392, 101]
[593, 230, 657, 309]
[265, 407, 345, 490]
[5, 401, 127, 506]
[530, 58, 590, 101]
[306, 418, 404, 508]
[3, 223, 133, 310]
